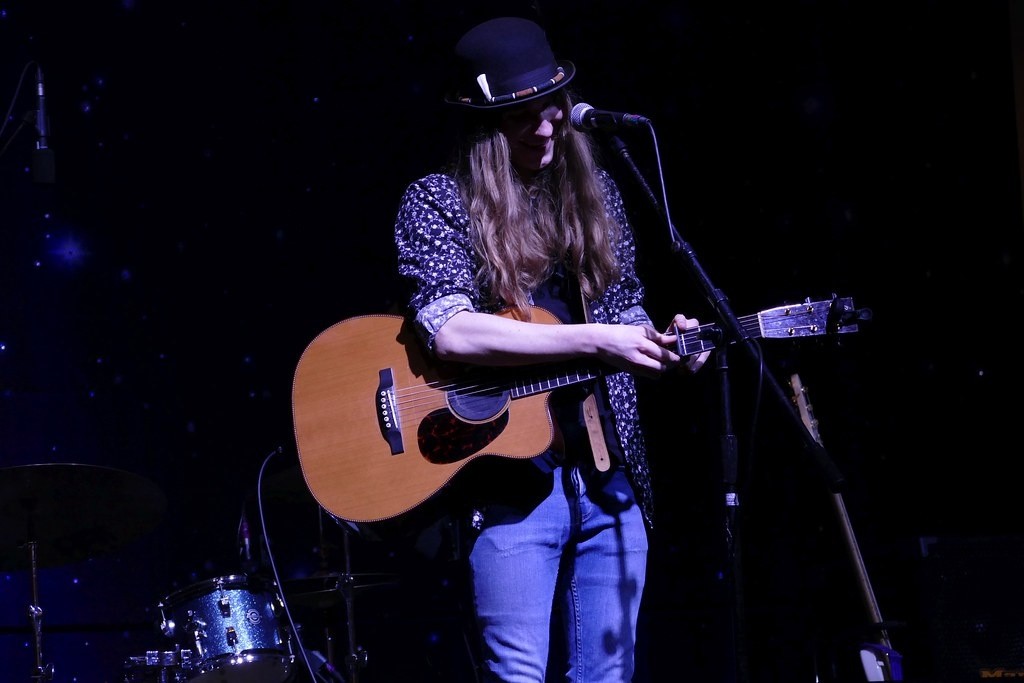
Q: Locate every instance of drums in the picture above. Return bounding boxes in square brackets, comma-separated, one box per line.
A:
[147, 571, 296, 683]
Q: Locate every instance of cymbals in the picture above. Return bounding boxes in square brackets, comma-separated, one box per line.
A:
[0, 461, 142, 509]
[289, 566, 387, 595]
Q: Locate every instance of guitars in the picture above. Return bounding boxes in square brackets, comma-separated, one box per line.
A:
[290, 291, 876, 525]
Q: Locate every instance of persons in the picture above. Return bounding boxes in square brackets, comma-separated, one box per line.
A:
[393, 18, 711, 683]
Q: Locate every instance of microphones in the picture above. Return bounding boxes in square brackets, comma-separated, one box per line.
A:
[568, 103, 651, 134]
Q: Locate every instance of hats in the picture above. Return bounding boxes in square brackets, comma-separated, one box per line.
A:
[443, 17, 576, 109]
